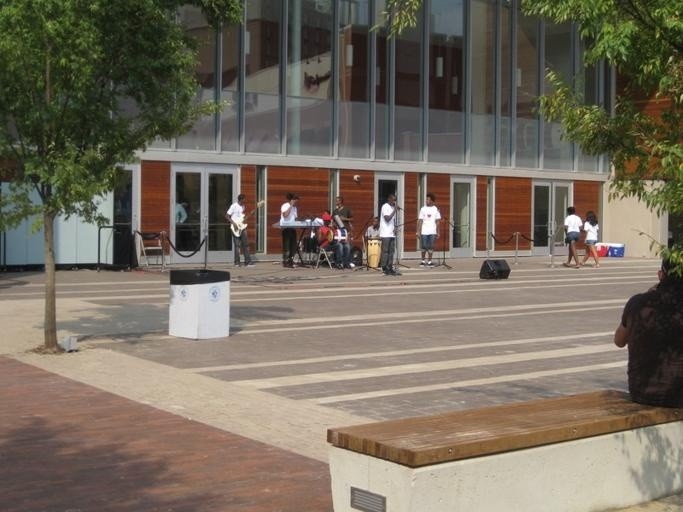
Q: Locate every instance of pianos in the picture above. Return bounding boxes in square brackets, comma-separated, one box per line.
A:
[280, 218, 324, 228]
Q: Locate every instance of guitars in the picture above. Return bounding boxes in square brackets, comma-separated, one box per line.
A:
[231, 201, 262, 238]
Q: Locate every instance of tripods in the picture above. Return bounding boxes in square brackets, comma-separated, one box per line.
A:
[391, 219, 415, 269]
[355, 217, 380, 272]
[431, 209, 456, 269]
[294, 215, 323, 269]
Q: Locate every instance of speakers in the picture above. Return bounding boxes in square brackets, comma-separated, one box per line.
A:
[479, 260, 510, 279]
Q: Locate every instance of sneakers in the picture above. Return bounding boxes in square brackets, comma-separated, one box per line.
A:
[420, 261, 435, 268]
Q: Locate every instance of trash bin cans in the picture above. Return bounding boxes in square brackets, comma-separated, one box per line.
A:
[168, 269, 231, 340]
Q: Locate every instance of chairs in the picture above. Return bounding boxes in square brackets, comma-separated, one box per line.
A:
[313, 239, 334, 269]
[137, 231, 166, 267]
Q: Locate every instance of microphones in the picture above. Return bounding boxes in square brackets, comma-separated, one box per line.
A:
[433, 203, 440, 209]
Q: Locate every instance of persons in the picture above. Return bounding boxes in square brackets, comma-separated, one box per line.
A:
[365, 217, 379, 238]
[175, 191, 190, 252]
[581, 211, 601, 268]
[416, 194, 442, 268]
[280, 194, 299, 268]
[225, 194, 256, 267]
[616, 259, 683, 407]
[332, 196, 354, 268]
[378, 194, 399, 274]
[318, 215, 355, 268]
[563, 207, 583, 268]
[325, 210, 330, 215]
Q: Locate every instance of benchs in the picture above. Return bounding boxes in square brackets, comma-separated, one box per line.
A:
[325, 388, 682, 511]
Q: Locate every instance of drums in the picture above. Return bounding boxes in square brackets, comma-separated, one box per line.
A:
[328, 228, 347, 244]
[367, 237, 382, 269]
